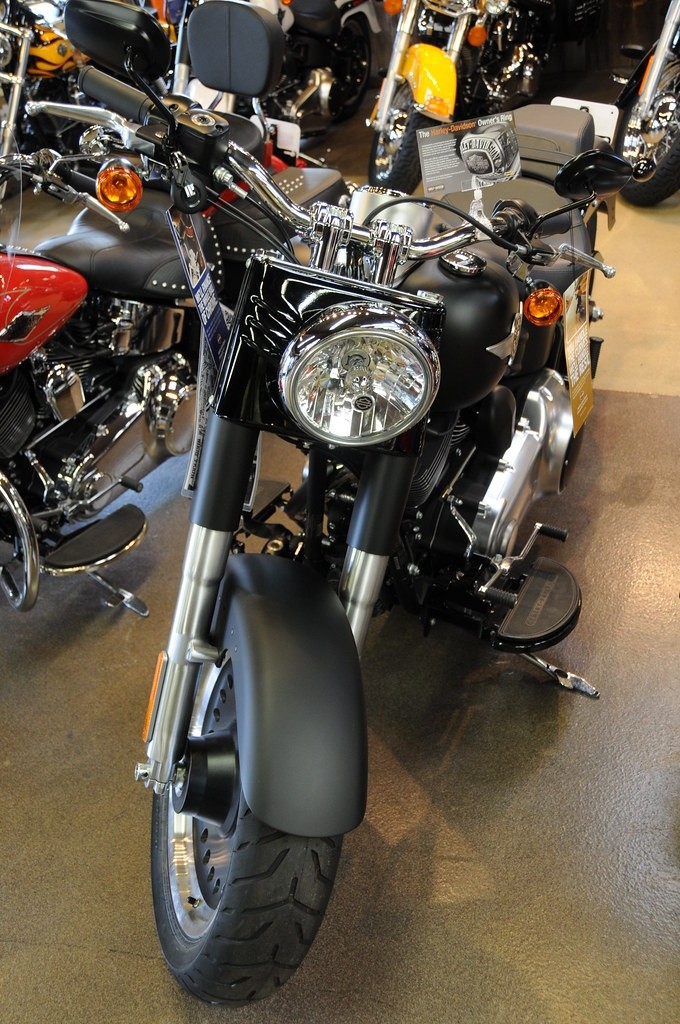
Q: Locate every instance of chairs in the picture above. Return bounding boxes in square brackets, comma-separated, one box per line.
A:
[147, 0, 287, 194]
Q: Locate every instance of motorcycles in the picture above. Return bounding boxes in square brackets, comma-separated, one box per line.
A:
[23, 0, 634, 1011]
[0, 0, 680, 618]
